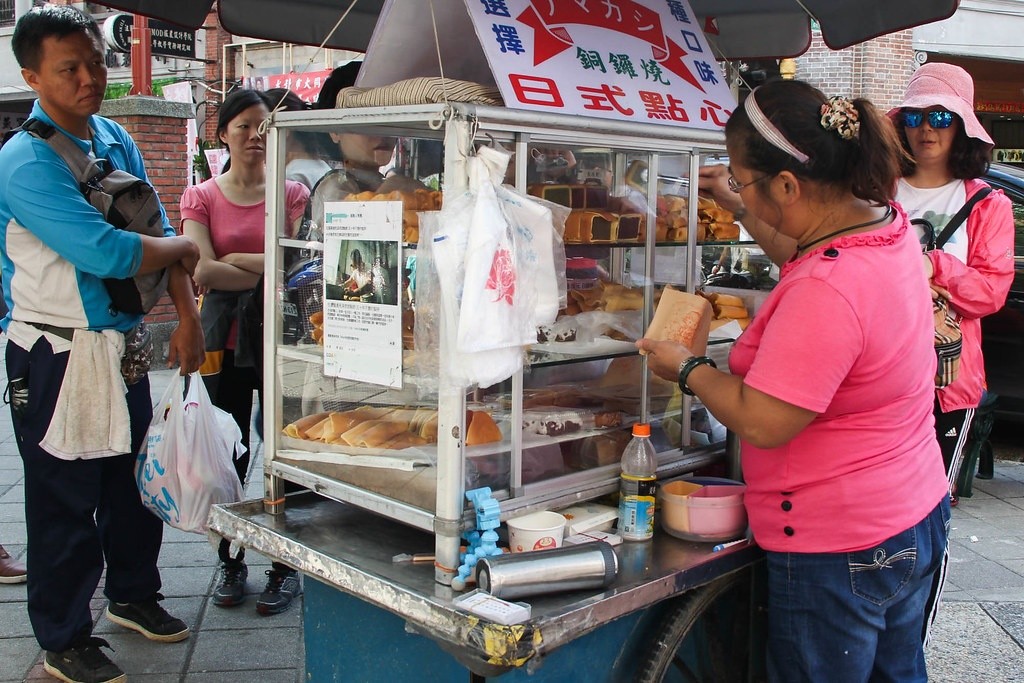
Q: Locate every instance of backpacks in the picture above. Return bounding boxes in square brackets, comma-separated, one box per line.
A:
[906, 187, 994, 387]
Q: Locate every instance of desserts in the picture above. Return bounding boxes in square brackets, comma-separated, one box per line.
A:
[343, 189, 442, 243]
[529, 182, 740, 243]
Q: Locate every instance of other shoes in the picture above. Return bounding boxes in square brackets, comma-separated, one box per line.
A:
[0, 545, 28, 583]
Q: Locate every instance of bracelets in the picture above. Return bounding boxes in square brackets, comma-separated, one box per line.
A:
[678, 356, 717, 396]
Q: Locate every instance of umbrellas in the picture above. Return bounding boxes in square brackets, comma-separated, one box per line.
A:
[85, 1, 958, 63]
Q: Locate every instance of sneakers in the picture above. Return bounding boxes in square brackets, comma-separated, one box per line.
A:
[211, 562, 248, 606]
[255, 567, 303, 613]
[106, 593, 190, 642]
[43, 637, 128, 683]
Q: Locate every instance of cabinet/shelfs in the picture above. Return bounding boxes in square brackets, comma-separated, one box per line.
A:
[263, 101, 780, 587]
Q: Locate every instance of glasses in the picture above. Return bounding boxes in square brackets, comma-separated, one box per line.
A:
[727, 166, 796, 193]
[900, 110, 956, 128]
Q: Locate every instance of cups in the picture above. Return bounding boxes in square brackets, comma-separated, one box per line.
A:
[506, 510, 566, 553]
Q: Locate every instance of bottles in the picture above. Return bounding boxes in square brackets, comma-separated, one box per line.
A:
[475, 541, 618, 599]
[617, 422, 657, 541]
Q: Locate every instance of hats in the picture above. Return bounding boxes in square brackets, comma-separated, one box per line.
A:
[884, 62, 995, 152]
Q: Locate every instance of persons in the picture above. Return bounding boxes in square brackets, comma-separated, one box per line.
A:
[633, 79, 953, 683]
[0, 7, 208, 683]
[264, 88, 333, 191]
[296, 61, 398, 412]
[182, 91, 313, 613]
[881, 62, 1015, 508]
[501, 143, 577, 188]
[0, 542, 27, 584]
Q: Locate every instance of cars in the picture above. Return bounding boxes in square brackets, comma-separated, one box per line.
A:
[960, 159, 1024, 438]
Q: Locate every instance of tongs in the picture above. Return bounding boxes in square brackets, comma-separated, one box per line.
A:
[640, 168, 689, 187]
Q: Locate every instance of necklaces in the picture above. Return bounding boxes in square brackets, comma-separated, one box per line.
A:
[798, 204, 892, 251]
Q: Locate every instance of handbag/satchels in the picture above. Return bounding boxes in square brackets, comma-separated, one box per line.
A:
[26, 321, 154, 384]
[134, 367, 247, 534]
[10, 117, 169, 318]
[243, 169, 367, 347]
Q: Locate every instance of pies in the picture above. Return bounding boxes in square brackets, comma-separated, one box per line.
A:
[279, 403, 503, 451]
[557, 280, 754, 331]
